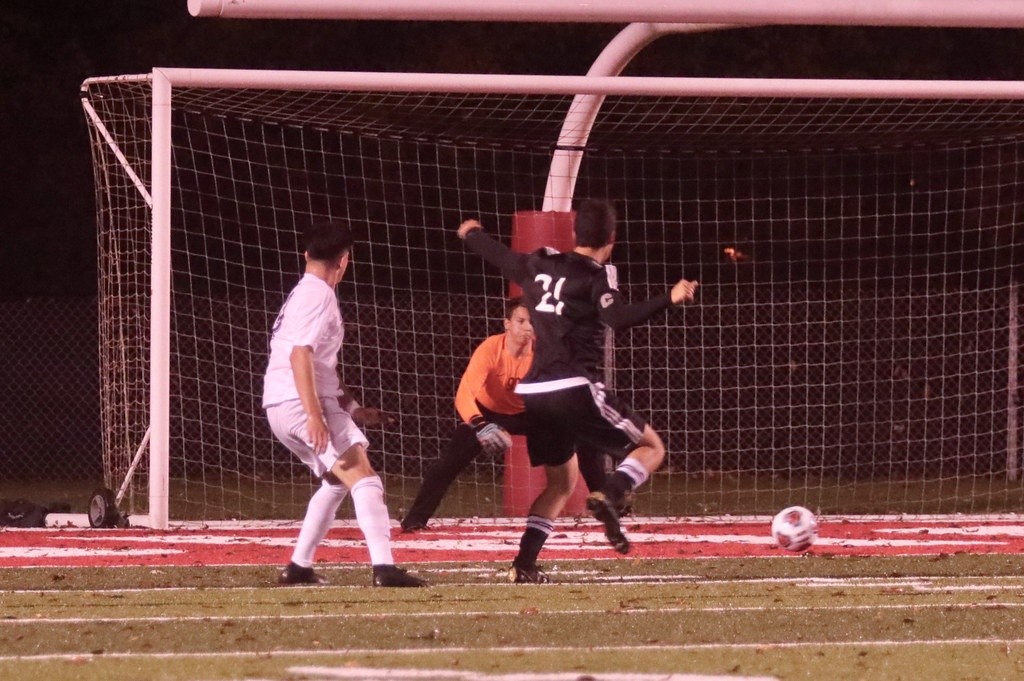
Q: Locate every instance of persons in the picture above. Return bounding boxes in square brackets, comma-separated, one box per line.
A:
[259, 220, 435, 588]
[457, 200, 698, 585]
[399, 294, 636, 533]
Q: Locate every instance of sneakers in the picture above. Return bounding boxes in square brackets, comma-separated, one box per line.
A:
[587, 484, 630, 555]
[370, 565, 429, 587]
[279, 569, 328, 584]
[508, 563, 550, 584]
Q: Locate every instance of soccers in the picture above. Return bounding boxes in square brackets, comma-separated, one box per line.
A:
[771, 504, 818, 551]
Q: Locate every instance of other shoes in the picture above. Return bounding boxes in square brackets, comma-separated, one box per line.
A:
[399, 520, 429, 534]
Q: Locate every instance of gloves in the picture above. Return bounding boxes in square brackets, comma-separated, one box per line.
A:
[476, 422, 512, 455]
